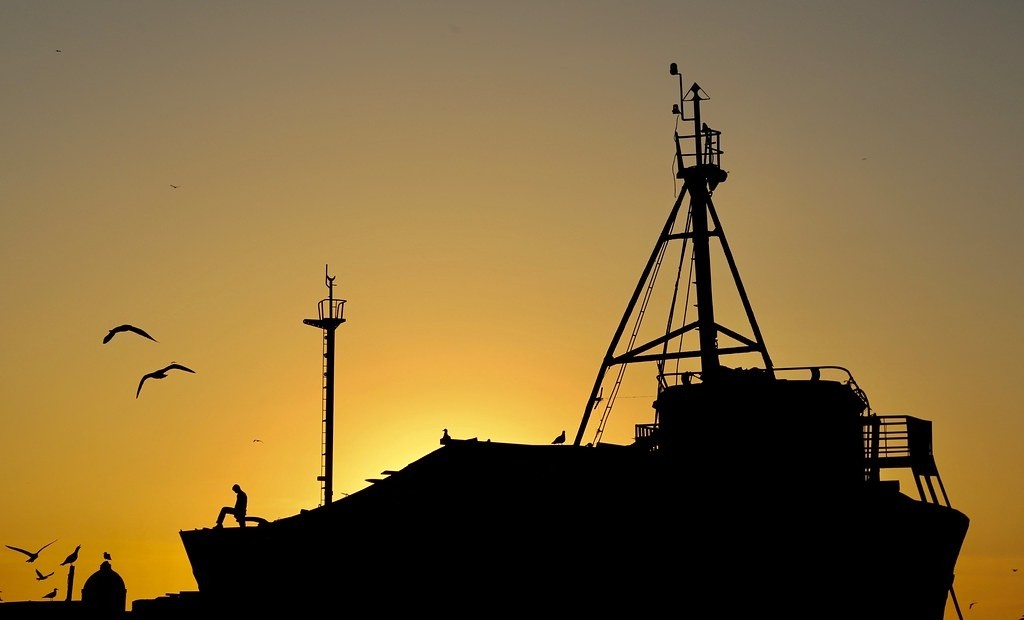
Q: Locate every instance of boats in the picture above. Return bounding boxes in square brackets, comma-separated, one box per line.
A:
[181, 63, 971, 620]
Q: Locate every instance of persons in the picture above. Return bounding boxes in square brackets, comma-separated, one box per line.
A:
[213, 484, 247, 529]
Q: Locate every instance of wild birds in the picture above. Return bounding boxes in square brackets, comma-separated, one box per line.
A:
[103, 551, 112, 562]
[42, 587, 59, 601]
[5, 536, 63, 564]
[35, 568, 55, 582]
[443, 428, 451, 439]
[135, 360, 196, 398]
[252, 439, 262, 443]
[59, 544, 82, 567]
[103, 324, 159, 345]
[551, 430, 566, 445]
